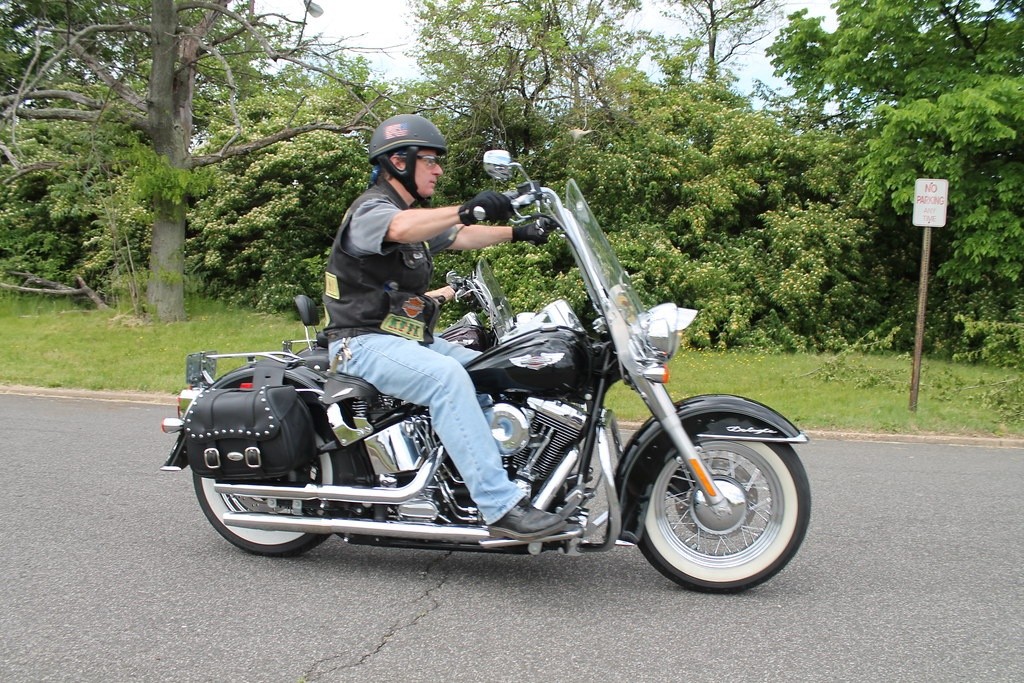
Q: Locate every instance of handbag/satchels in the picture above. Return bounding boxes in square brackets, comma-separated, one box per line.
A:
[183, 384, 316, 482]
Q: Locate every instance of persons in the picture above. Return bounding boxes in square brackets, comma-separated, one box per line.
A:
[323, 113, 568, 540]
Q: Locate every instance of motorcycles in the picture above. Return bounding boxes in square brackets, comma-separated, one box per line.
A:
[159, 149, 813, 596]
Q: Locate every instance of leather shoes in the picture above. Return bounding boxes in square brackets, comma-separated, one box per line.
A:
[488, 496, 568, 540]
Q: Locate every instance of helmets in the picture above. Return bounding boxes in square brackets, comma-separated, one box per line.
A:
[368, 114, 447, 166]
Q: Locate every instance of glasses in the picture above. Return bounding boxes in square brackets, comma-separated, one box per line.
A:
[395, 150, 442, 164]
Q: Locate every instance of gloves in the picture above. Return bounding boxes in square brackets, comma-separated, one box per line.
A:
[511, 217, 558, 247]
[458, 190, 513, 227]
